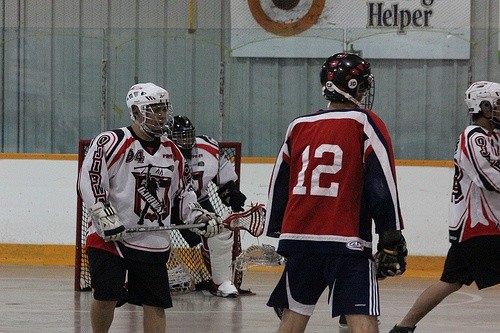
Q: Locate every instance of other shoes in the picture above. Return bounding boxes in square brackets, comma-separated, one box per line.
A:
[209, 283, 237, 298]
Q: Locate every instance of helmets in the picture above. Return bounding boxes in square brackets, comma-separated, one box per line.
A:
[464, 81, 500, 113]
[126, 83, 170, 121]
[320, 53, 370, 102]
[166, 116, 193, 134]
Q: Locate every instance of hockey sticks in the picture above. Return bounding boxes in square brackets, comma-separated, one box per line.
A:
[125, 205, 267, 237]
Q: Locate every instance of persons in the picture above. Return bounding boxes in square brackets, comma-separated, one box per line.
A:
[75, 82, 225, 333]
[264, 52, 409, 333]
[387, 80, 500, 333]
[164, 115, 248, 299]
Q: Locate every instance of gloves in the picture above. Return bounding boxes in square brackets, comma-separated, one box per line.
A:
[186, 209, 224, 238]
[90, 201, 126, 242]
[377, 231, 408, 279]
[217, 180, 246, 212]
